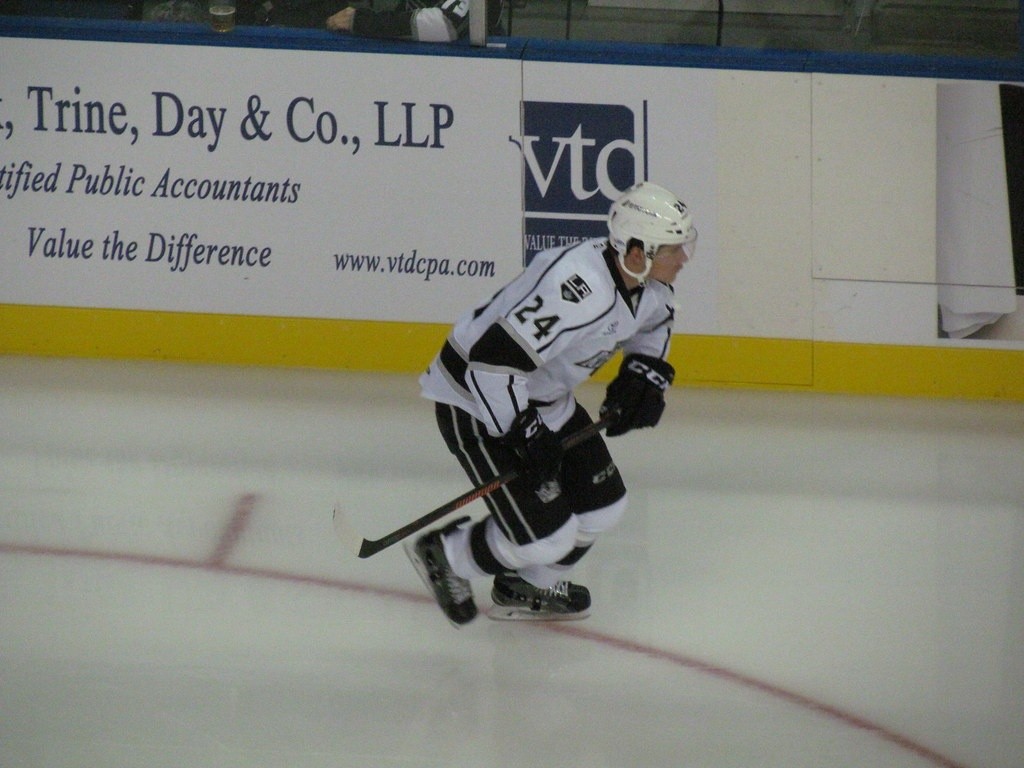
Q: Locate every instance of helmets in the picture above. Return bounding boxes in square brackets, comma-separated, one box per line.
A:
[607, 182, 698, 258]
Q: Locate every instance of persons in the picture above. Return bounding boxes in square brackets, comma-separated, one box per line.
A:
[401, 180, 699, 631]
[325, 0, 504, 44]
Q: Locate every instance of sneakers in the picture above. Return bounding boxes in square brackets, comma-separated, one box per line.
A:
[401, 516, 479, 624]
[486, 571, 592, 620]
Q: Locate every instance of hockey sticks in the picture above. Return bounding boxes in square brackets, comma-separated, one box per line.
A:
[331, 410, 622, 562]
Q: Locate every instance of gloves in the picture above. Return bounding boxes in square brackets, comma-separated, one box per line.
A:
[599, 351, 675, 436]
[505, 406, 563, 488]
